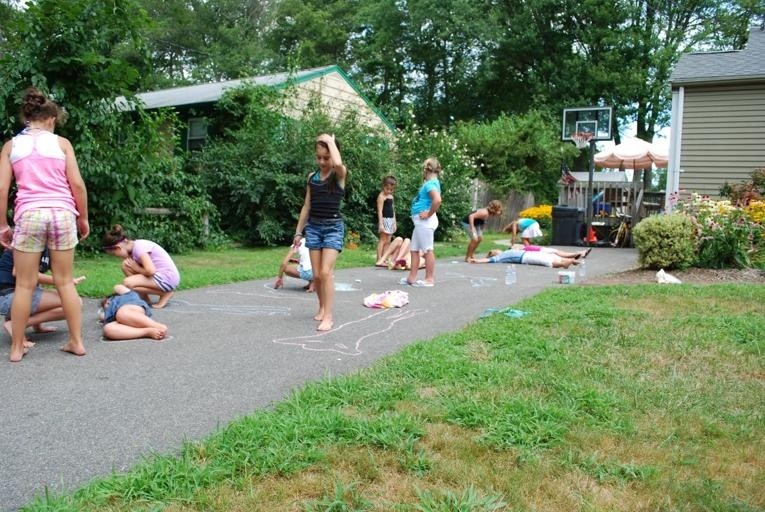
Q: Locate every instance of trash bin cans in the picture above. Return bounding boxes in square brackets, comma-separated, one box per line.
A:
[552, 204, 577, 247]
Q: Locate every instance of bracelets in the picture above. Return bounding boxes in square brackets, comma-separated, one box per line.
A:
[294, 233, 303, 238]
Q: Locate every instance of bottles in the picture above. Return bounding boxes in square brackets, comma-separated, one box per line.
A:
[506, 263, 516, 284]
[579, 260, 586, 277]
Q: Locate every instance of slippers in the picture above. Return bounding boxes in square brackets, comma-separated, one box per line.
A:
[399, 278, 434, 287]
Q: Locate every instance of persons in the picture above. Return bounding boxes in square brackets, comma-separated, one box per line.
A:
[397, 157, 443, 289]
[292, 133, 347, 332]
[374, 176, 594, 287]
[1, 245, 87, 349]
[0, 85, 91, 362]
[0, 225, 11, 234]
[274, 224, 316, 293]
[96, 282, 169, 341]
[100, 222, 181, 308]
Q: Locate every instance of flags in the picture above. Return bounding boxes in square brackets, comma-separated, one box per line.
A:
[562, 154, 577, 186]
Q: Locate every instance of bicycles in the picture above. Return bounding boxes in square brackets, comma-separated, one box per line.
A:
[608, 213, 632, 248]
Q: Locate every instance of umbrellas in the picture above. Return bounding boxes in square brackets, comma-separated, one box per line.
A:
[591, 130, 672, 184]
